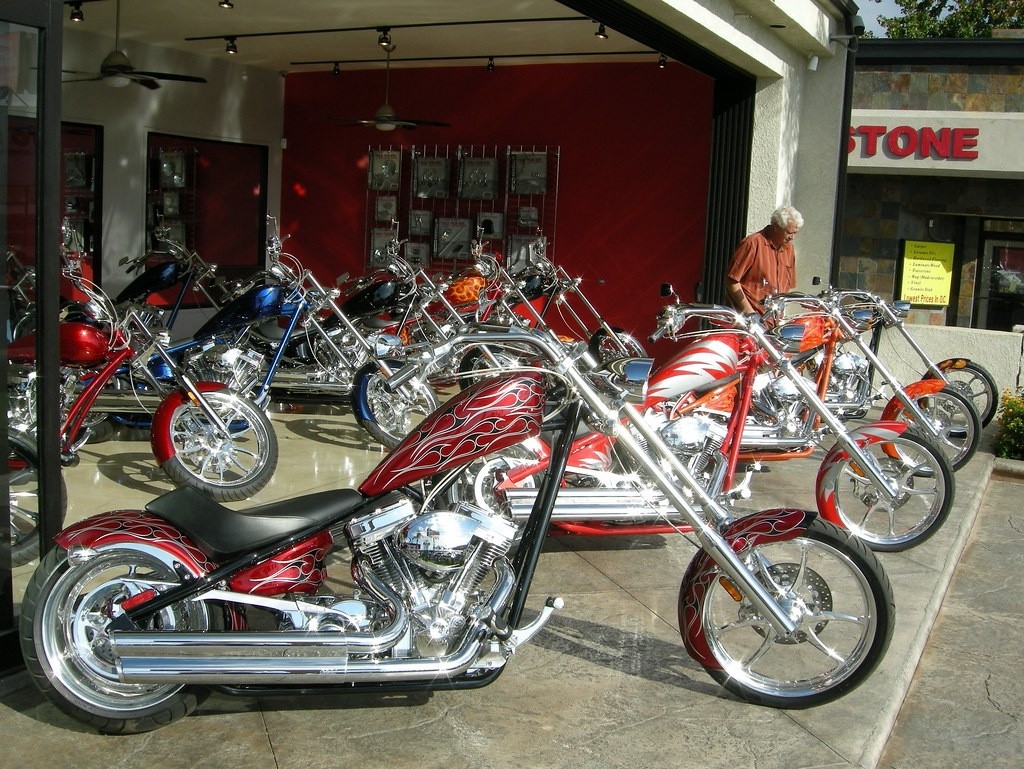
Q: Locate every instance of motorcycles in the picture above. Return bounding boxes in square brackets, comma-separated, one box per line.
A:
[433, 281, 956, 552]
[589, 277, 998, 477]
[7, 216, 649, 571]
[17, 315, 895, 734]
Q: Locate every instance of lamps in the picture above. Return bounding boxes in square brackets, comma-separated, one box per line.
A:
[487, 56, 495, 72]
[806, 49, 819, 72]
[594, 23, 609, 39]
[70, 0, 85, 22]
[332, 61, 343, 78]
[375, 123, 396, 132]
[101, 76, 131, 88]
[375, 27, 391, 46]
[224, 36, 238, 54]
[659, 54, 668, 68]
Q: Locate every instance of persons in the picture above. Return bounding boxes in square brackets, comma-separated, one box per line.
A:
[724, 207, 804, 330]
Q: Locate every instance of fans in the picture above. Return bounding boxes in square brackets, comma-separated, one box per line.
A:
[30, 0, 208, 91]
[324, 46, 452, 132]
